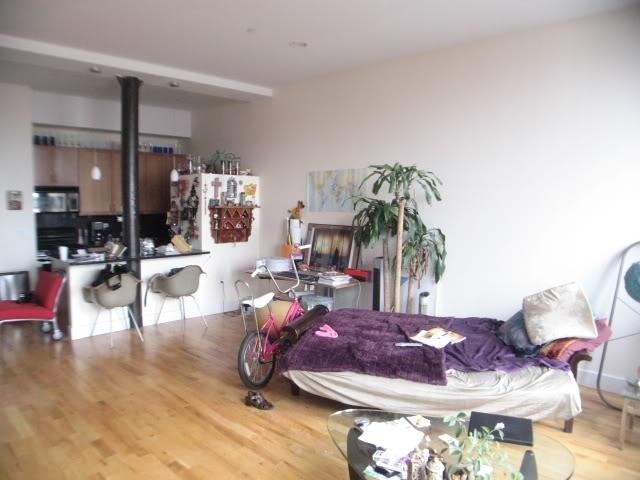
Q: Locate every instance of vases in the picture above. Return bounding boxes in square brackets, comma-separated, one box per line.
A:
[286, 216, 302, 245]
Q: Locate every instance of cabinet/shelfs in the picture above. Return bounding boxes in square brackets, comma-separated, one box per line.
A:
[33, 144, 190, 216]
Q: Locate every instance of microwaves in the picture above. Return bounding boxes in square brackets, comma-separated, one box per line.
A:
[33, 192, 79, 213]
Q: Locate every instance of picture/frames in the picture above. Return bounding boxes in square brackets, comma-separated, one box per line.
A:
[303, 221, 361, 274]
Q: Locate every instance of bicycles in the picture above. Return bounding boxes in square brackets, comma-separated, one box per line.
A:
[235, 243, 313, 390]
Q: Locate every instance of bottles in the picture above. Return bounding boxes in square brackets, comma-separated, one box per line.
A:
[425, 456, 446, 480]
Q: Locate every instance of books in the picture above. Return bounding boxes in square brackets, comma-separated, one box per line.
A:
[409, 327, 466, 350]
[467, 411, 533, 447]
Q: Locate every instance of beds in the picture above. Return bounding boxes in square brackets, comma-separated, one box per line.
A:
[274, 308, 595, 437]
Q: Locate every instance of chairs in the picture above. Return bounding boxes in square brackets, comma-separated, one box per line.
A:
[80, 271, 147, 347]
[149, 264, 213, 331]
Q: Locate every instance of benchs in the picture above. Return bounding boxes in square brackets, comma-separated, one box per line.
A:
[0, 270, 70, 344]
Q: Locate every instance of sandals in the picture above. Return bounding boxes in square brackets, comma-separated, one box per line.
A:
[245, 390, 274, 411]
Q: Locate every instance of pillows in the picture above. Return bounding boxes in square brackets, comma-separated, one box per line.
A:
[522, 282, 600, 344]
[493, 308, 541, 360]
[534, 316, 615, 365]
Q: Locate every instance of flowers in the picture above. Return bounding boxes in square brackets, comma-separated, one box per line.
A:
[287, 198, 307, 219]
[436, 409, 525, 479]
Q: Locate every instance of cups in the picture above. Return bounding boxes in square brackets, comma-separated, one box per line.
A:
[188, 160, 193, 174]
[58, 246, 69, 262]
[144, 144, 174, 153]
[220, 160, 241, 174]
[32, 134, 56, 146]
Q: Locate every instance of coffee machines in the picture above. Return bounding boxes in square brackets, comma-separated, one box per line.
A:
[90, 221, 110, 247]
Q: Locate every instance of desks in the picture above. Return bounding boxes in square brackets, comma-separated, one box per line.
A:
[245, 262, 375, 310]
[613, 384, 640, 451]
[44, 242, 215, 343]
